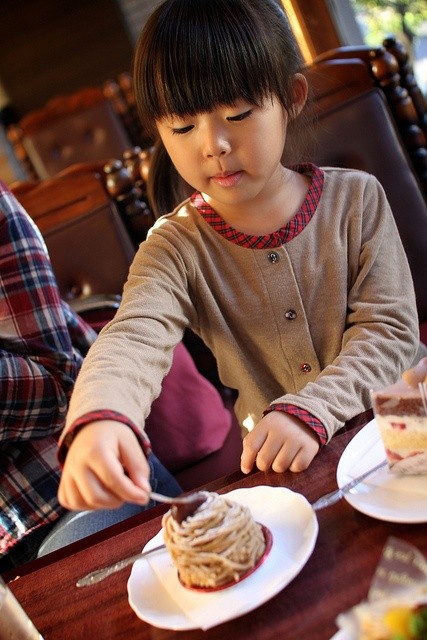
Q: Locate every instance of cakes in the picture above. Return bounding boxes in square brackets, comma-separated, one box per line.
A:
[163, 489, 276, 591]
[368, 354, 427, 477]
[359, 603, 426, 638]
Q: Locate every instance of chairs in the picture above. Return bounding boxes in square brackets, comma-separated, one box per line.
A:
[24, 103, 135, 182]
[163, 49, 426, 404]
[0, 159, 230, 405]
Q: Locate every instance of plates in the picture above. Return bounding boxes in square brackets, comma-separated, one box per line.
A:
[336, 418, 427, 524]
[127, 485, 320, 632]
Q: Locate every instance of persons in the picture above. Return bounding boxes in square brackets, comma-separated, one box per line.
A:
[53, 0, 423, 512]
[1, 182, 189, 579]
